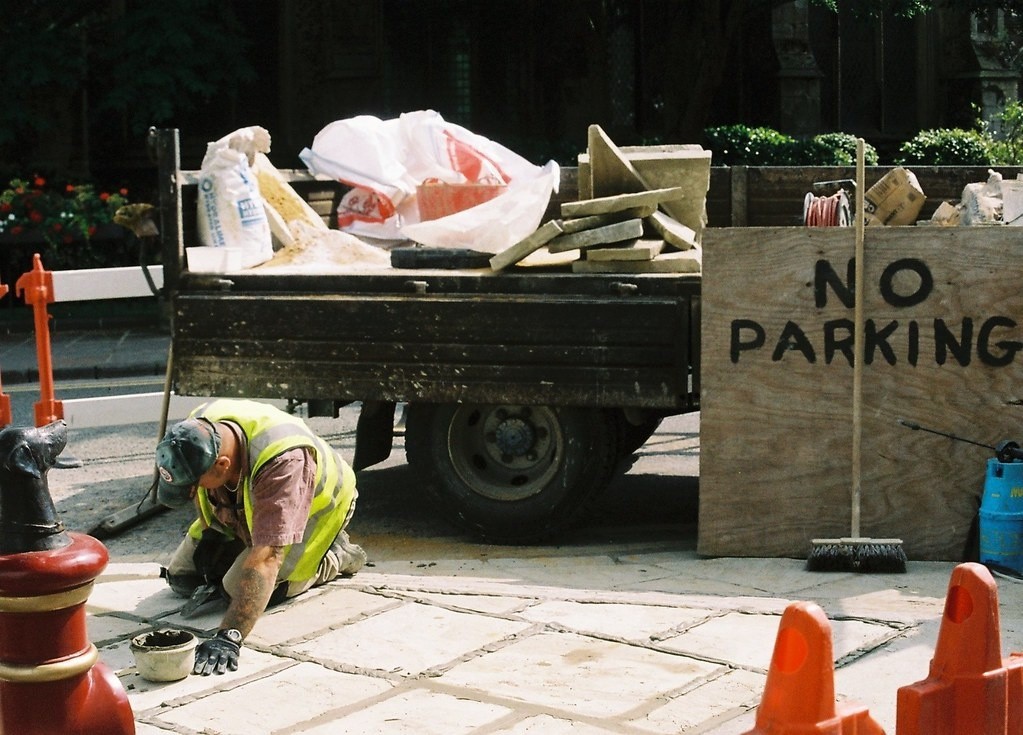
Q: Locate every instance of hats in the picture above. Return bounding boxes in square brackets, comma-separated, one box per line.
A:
[154, 416, 221, 510]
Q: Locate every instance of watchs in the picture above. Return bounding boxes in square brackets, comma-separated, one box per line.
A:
[216, 628, 243, 649]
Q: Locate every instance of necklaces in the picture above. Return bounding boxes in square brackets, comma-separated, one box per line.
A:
[223, 467, 242, 492]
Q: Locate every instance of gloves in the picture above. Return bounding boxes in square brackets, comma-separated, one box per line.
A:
[194, 627, 244, 676]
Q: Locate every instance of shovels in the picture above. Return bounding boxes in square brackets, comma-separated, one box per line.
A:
[178, 551, 220, 616]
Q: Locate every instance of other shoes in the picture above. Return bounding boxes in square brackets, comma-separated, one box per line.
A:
[330, 529, 367, 576]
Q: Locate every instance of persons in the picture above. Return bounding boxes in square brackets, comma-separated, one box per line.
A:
[155, 397, 367, 676]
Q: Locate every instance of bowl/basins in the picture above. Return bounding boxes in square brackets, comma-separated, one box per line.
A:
[130, 630, 198, 682]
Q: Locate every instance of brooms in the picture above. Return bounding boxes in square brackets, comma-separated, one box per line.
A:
[803, 139, 911, 577]
[88, 338, 171, 541]
